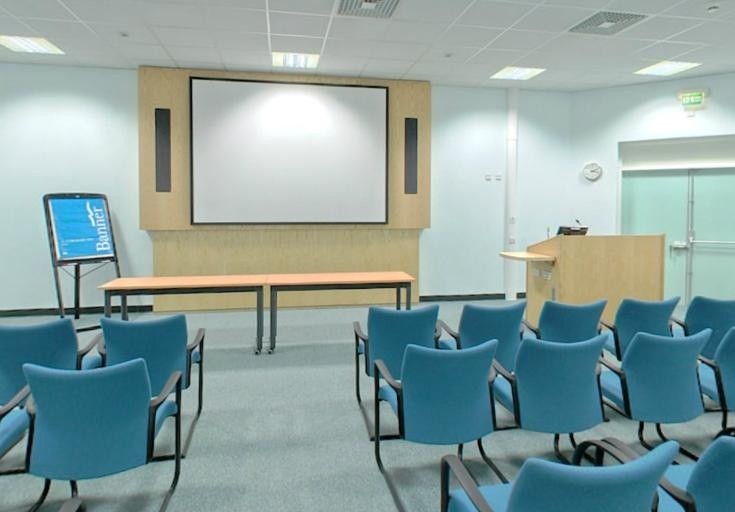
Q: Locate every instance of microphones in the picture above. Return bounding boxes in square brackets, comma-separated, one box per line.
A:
[575, 219, 583, 228]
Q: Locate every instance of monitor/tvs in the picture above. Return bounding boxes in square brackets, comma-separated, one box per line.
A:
[557, 226, 589, 235]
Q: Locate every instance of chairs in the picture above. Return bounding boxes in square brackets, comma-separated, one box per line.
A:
[2, 379, 37, 476]
[23, 356, 183, 510]
[353, 295, 735, 511]
[48, 493, 89, 510]
[1, 315, 103, 409]
[98, 315, 204, 416]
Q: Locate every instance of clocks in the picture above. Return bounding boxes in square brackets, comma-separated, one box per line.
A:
[581, 163, 603, 180]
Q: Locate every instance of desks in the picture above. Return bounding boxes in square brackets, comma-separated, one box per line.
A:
[266, 272, 418, 352]
[97, 274, 264, 354]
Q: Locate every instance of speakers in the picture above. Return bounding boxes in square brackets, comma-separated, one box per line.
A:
[404, 117, 418, 194]
[155, 107, 172, 192]
